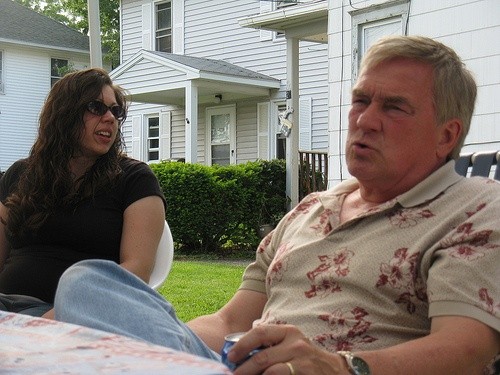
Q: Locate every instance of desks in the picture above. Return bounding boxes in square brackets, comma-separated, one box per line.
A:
[0, 310, 231, 375]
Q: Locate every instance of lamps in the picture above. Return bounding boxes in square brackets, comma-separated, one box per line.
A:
[213, 93, 224, 104]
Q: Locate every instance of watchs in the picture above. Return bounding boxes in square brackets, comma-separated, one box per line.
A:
[336, 349, 370, 375]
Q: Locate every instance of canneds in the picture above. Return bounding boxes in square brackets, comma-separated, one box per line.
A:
[220, 332, 273, 375]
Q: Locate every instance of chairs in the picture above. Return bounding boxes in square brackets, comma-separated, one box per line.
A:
[148, 220, 174, 292]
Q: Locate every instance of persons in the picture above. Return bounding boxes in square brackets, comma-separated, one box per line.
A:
[52, 33, 500, 374]
[1, 69, 169, 320]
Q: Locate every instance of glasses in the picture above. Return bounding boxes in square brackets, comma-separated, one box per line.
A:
[86, 100, 126, 121]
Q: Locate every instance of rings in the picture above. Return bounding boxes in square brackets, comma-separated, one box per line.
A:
[286, 361, 296, 374]
